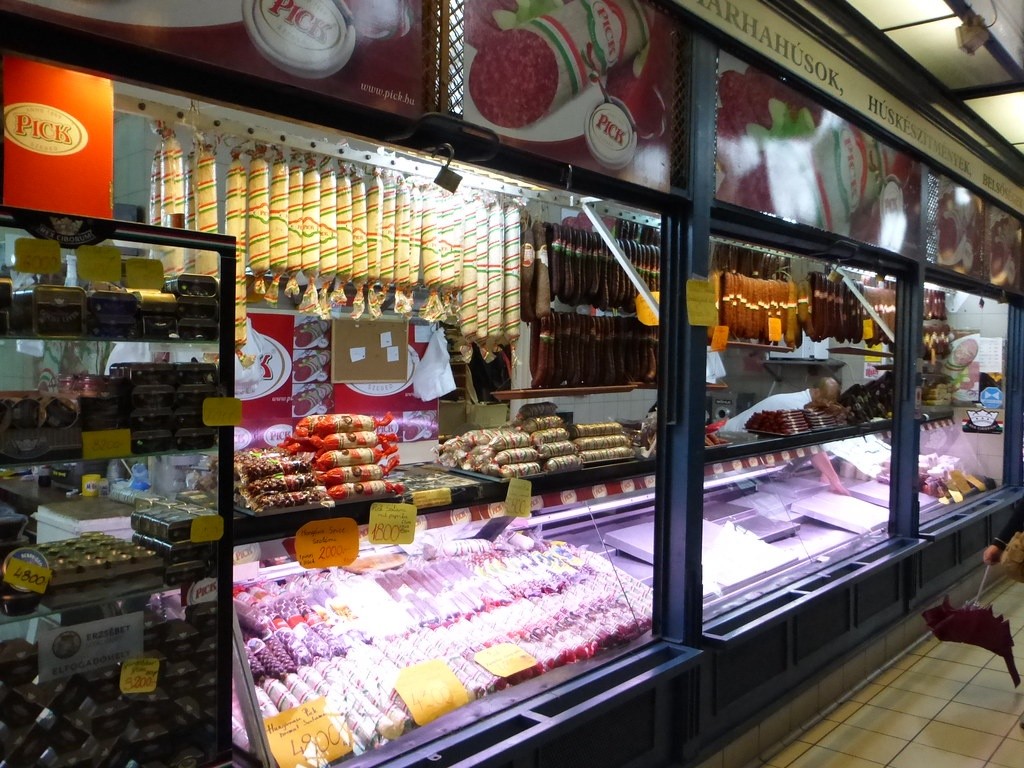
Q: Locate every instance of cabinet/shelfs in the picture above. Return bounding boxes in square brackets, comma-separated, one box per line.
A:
[0, 204, 235, 768]
[234, 406, 994, 768]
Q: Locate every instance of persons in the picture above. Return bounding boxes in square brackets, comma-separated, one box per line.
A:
[984, 496, 1024, 567]
[714, 376, 851, 498]
